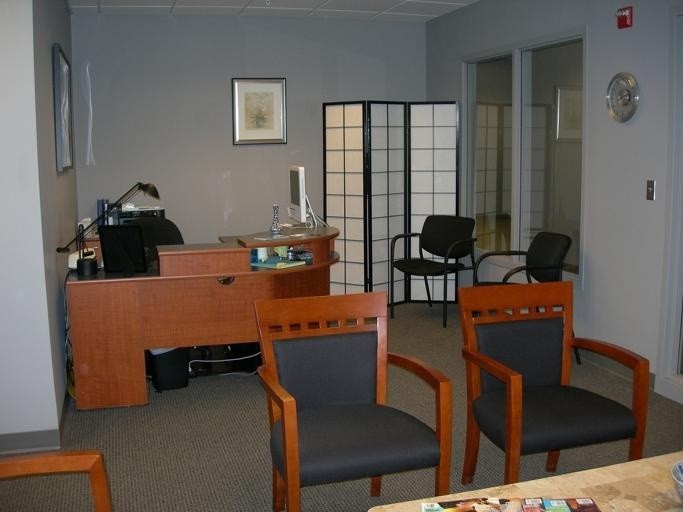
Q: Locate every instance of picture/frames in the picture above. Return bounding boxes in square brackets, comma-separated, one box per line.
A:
[51, 41, 74, 176]
[552, 85, 584, 145]
[230, 77, 288, 146]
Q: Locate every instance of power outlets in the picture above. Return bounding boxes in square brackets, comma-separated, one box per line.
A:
[647, 178, 655, 201]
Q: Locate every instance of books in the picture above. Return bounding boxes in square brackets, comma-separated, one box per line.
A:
[97, 198, 118, 231]
[250, 260, 306, 269]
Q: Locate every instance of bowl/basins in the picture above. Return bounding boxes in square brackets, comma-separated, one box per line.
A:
[672, 461, 683, 502]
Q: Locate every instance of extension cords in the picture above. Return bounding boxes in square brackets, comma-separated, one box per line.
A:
[183, 367, 196, 377]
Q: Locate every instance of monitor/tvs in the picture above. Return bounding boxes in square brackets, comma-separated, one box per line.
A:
[287, 166, 306, 224]
[97, 225, 147, 273]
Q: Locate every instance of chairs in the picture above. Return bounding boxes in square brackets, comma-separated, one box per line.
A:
[120, 218, 184, 245]
[458, 280, 650, 489]
[252, 291, 451, 510]
[0, 451, 113, 512]
[473, 231, 582, 367]
[389, 214, 479, 328]
[497, 212, 511, 251]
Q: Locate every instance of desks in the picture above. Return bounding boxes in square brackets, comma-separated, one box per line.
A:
[65, 227, 341, 410]
[367, 450, 683, 512]
[165, 300, 172, 302]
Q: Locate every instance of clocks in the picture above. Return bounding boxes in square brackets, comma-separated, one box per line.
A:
[606, 73, 642, 127]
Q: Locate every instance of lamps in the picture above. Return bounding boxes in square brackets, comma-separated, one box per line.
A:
[57, 182, 160, 270]
